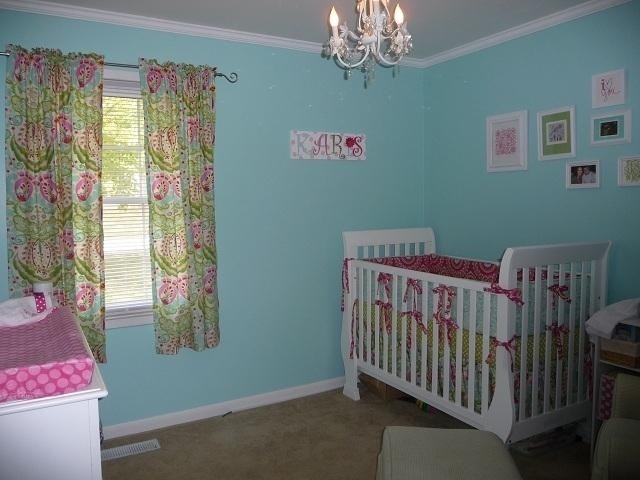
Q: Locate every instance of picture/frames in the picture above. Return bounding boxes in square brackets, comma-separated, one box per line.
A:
[485, 111, 528, 172]
[536, 105, 576, 160]
[565, 160, 599, 188]
[591, 68, 626, 108]
[618, 156, 640, 187]
[590, 110, 632, 146]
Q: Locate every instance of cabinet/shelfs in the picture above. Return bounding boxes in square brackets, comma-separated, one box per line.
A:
[589, 316, 640, 474]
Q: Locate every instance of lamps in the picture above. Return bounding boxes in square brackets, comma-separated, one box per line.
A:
[321, 0, 415, 82]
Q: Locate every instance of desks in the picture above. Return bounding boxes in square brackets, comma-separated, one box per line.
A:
[0, 305, 109, 480]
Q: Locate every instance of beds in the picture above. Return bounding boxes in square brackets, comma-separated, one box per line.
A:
[340, 228, 613, 447]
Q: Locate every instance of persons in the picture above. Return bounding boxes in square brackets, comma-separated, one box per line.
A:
[571, 165, 596, 184]
[548, 124, 564, 142]
